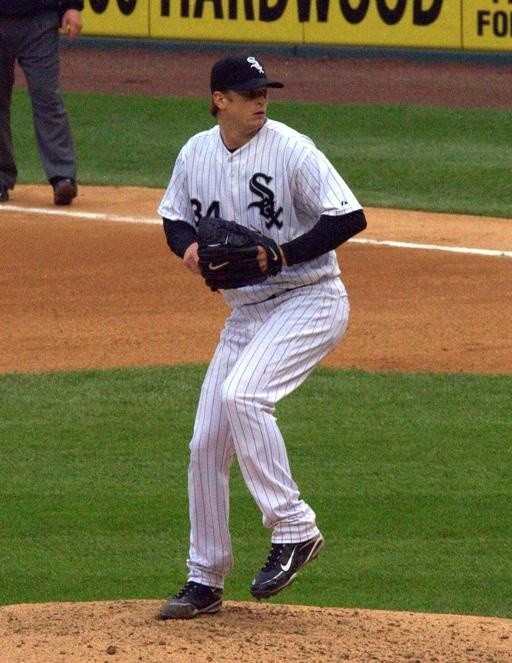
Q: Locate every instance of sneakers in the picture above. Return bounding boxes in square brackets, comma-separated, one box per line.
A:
[249, 531, 324, 601]
[159, 581, 222, 620]
[53, 175, 77, 206]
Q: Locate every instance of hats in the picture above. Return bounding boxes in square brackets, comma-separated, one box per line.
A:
[210, 54, 284, 90]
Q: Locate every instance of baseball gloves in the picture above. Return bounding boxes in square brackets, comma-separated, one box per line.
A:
[196, 217, 281, 290]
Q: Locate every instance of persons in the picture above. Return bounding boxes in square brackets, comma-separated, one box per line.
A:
[157, 54, 367, 617]
[0, 0, 84, 205]
